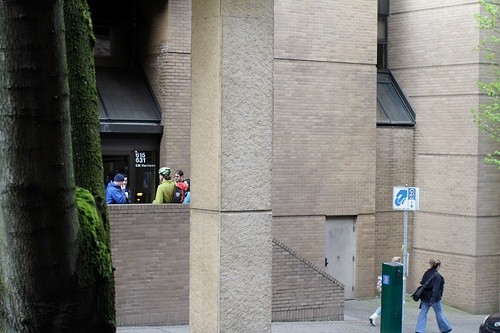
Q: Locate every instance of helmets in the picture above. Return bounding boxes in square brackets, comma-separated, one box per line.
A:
[159, 167, 170, 176]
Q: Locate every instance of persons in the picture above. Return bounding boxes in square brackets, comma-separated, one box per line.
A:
[370, 257, 402, 326]
[416, 258, 452, 333]
[104, 174, 131, 205]
[152, 167, 190, 204]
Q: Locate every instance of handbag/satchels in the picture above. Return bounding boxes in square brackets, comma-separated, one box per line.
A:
[411, 286, 423, 302]
[173, 185, 185, 202]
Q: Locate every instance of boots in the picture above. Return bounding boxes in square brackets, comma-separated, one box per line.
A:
[369, 313, 379, 326]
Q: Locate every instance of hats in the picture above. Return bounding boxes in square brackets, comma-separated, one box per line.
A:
[114, 174, 124, 181]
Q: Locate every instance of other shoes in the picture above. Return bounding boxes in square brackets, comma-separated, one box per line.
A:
[443, 328, 452, 333]
